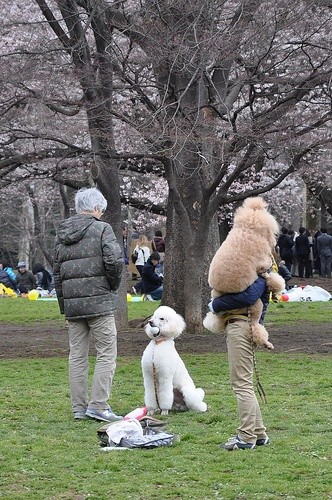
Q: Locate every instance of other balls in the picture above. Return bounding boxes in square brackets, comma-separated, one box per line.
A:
[281, 294, 290, 302]
[149, 327, 160, 335]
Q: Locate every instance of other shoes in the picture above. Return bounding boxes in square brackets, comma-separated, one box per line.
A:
[299, 275, 304, 278]
[305, 276, 312, 278]
[132, 286, 138, 295]
[147, 294, 154, 301]
[291, 275, 298, 278]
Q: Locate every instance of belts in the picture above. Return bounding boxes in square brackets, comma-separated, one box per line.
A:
[225, 319, 246, 327]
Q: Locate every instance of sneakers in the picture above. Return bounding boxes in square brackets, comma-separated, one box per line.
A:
[221, 436, 258, 451]
[227, 436, 270, 445]
[85, 407, 123, 423]
[74, 409, 85, 419]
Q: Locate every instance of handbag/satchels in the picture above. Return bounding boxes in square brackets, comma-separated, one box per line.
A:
[157, 252, 165, 264]
[131, 250, 138, 264]
[119, 432, 180, 449]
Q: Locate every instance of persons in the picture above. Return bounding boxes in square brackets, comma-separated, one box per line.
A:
[123, 224, 332, 301]
[209, 250, 276, 450]
[53, 187, 124, 421]
[0, 262, 55, 299]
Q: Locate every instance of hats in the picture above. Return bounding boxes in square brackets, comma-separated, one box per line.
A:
[16, 261, 25, 267]
[122, 231, 127, 235]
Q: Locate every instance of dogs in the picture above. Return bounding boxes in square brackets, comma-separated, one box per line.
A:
[201, 195, 285, 350]
[140, 305, 208, 416]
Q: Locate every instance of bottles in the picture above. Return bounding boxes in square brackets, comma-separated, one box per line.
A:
[123, 406, 147, 420]
